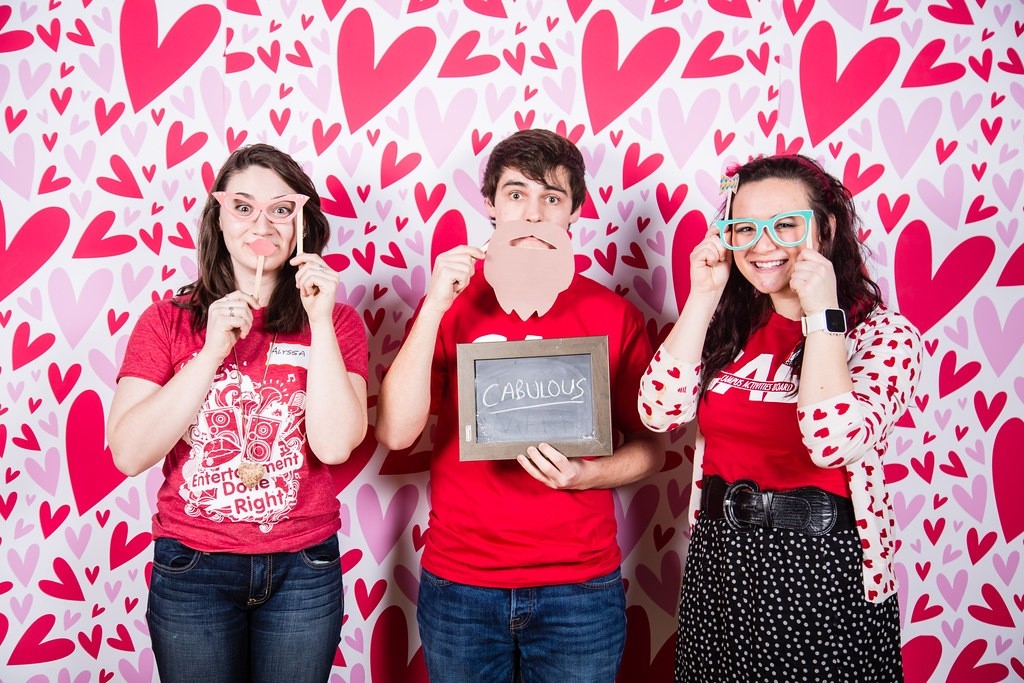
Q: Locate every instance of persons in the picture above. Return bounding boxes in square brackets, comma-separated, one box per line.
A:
[106, 145, 368, 683]
[638, 154, 922, 683]
[373, 129, 668, 683]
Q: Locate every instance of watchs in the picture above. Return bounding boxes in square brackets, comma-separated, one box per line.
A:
[801, 308, 847, 336]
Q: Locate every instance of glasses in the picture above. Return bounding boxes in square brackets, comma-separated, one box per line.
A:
[211, 191, 311, 224]
[716, 210, 813, 250]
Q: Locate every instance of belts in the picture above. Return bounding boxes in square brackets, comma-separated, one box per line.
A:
[700, 475, 856, 536]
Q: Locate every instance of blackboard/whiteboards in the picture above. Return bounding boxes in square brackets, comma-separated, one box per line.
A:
[456, 336, 611, 460]
